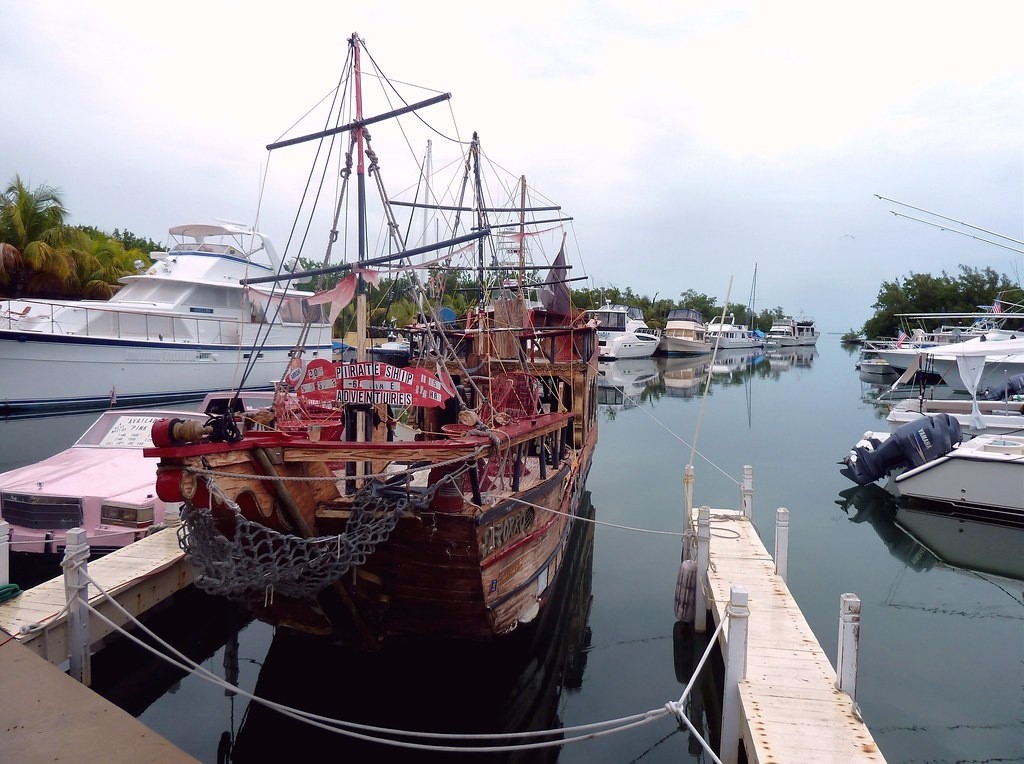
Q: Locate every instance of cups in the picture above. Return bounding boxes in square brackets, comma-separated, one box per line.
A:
[309, 425, 321, 442]
[543, 404, 551, 414]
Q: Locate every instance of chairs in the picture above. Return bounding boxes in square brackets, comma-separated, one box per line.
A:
[274, 359, 345, 469]
[440, 307, 588, 492]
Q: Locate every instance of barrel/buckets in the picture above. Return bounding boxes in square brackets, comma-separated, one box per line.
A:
[426, 465, 465, 513]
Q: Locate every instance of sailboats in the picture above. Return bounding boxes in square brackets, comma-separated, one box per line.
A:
[743, 261, 782, 348]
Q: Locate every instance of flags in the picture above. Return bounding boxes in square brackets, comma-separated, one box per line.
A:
[541, 245, 570, 314]
[993, 295, 1001, 313]
[897, 330, 906, 348]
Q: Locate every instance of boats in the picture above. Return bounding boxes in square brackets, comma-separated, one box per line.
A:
[0, 219, 333, 475]
[143, 25, 598, 636]
[836, 404, 1024, 521]
[855, 276, 1024, 440]
[583, 298, 662, 359]
[0, 384, 330, 589]
[656, 305, 713, 354]
[766, 312, 820, 347]
[706, 312, 754, 348]
